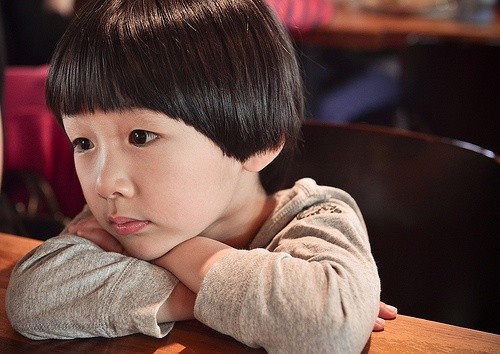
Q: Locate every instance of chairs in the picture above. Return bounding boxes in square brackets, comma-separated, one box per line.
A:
[257, 122, 499, 337]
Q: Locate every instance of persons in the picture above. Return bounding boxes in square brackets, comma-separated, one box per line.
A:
[4, 0, 400, 353]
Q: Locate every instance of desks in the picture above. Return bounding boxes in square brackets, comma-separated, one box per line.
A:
[0, 233, 500, 354]
